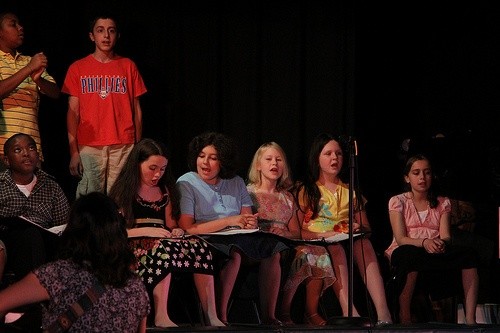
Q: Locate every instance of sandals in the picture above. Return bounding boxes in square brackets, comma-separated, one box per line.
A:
[303, 312, 326, 328]
[280, 313, 295, 328]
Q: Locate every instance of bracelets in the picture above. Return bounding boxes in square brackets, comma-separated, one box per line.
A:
[26, 64, 32, 74]
[422, 238, 427, 247]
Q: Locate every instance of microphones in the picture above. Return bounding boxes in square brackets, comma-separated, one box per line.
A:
[337, 135, 355, 140]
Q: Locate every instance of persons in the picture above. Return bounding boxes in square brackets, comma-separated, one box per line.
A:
[61, 13, 148, 207]
[0, 13, 59, 172]
[0, 191, 151, 333]
[0, 133, 392, 328]
[384, 154, 479, 325]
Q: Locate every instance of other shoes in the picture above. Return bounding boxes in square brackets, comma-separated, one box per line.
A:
[159, 320, 185, 333]
[262, 315, 283, 330]
[376, 320, 392, 329]
[218, 316, 231, 331]
